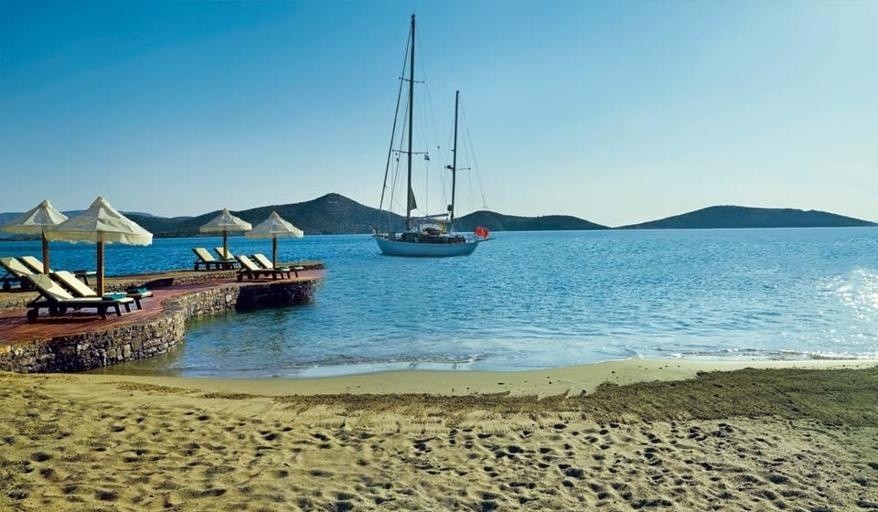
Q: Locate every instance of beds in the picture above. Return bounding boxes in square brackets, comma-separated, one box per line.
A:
[43, 195, 154, 298]
[199, 208, 253, 260]
[243, 210, 304, 268]
[0, 197, 68, 275]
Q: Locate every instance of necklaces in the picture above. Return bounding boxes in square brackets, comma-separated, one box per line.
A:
[369, 11, 498, 260]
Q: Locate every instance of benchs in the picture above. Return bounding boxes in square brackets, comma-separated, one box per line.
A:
[474, 226, 489, 239]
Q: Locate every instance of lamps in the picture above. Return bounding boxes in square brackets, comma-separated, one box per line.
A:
[50, 271, 153, 312]
[214, 247, 256, 269]
[21, 273, 135, 319]
[0, 257, 76, 290]
[251, 253, 304, 278]
[18, 256, 97, 285]
[234, 255, 290, 279]
[191, 248, 237, 270]
